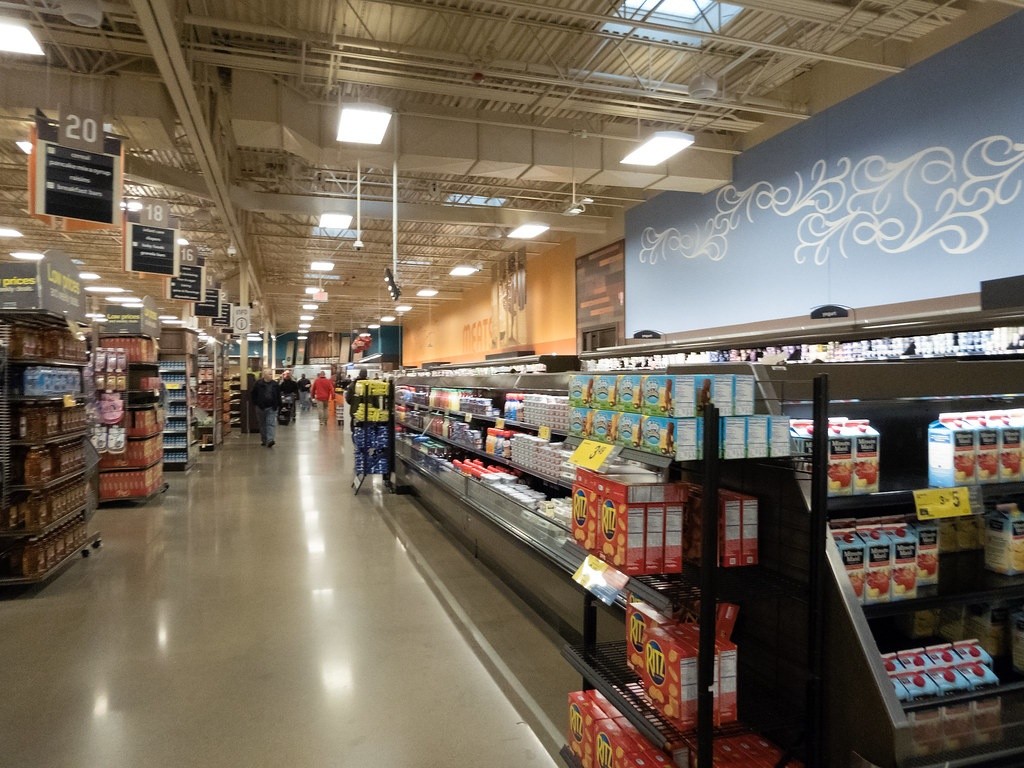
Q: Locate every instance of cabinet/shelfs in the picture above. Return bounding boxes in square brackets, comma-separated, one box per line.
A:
[222, 343, 232, 438]
[94, 322, 169, 504]
[393, 360, 829, 768]
[230, 355, 260, 428]
[751, 359, 1024, 768]
[197, 335, 225, 451]
[157, 327, 199, 472]
[0, 307, 101, 590]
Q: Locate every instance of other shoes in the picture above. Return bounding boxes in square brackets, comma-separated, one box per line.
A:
[268, 441, 275, 447]
[261, 442, 266, 446]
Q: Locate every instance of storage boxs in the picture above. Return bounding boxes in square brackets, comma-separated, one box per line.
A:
[395, 373, 803, 768]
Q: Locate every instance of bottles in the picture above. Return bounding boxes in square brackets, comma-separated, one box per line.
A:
[2, 319, 91, 577]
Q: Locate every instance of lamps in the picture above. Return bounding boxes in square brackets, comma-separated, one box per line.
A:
[448, 264, 476, 277]
[335, 103, 392, 146]
[618, 131, 696, 166]
[417, 289, 439, 297]
[318, 211, 353, 229]
[311, 261, 334, 271]
[506, 220, 550, 240]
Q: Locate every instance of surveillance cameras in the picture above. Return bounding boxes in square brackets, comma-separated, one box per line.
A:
[354, 241, 365, 250]
[227, 248, 237, 256]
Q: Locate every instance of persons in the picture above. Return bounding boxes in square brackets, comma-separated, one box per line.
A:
[252, 368, 380, 448]
